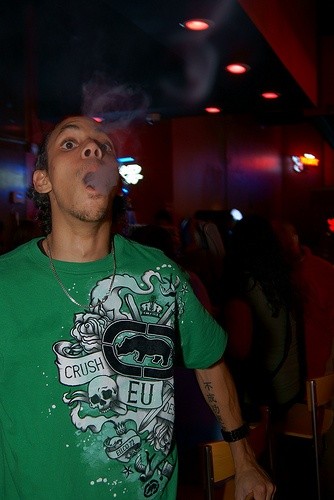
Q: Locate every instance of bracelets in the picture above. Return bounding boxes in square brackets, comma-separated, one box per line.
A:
[221, 421, 250, 442]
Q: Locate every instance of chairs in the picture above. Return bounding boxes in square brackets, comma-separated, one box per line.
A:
[276, 374, 334, 500]
[204, 405, 280, 500]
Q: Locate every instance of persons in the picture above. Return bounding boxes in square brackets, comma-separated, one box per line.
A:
[0, 116, 276, 500]
[137, 208, 334, 500]
[111, 195, 128, 234]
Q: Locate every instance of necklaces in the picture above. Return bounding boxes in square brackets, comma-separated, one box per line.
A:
[44, 237, 116, 308]
